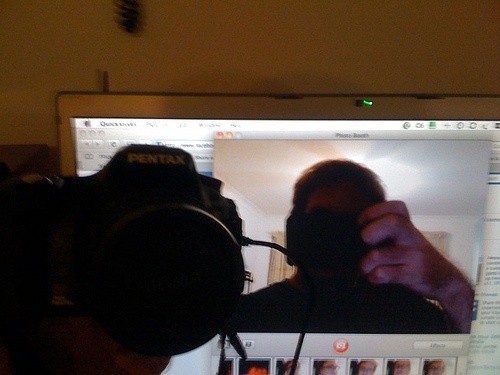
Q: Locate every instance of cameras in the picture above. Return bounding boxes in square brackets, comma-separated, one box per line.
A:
[1, 144, 249, 358]
[282, 205, 379, 267]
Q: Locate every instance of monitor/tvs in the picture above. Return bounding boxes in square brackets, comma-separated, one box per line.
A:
[55, 92, 500, 375]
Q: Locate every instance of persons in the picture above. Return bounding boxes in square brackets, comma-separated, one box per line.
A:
[356, 359, 377, 375]
[427, 360, 445, 375]
[281, 361, 300, 375]
[1, 171, 171, 375]
[313, 361, 338, 375]
[391, 359, 411, 375]
[237, 159, 473, 334]
[241, 360, 268, 375]
